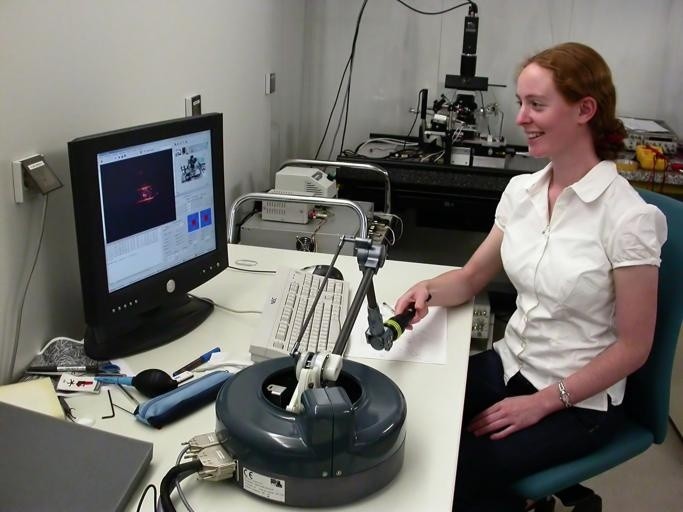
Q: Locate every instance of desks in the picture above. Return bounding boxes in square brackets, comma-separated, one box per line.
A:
[339, 133, 551, 214]
[0, 241, 475, 511]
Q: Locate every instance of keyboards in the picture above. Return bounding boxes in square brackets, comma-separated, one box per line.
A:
[249, 267, 349, 364]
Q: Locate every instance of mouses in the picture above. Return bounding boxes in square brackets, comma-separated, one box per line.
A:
[300, 265, 343, 280]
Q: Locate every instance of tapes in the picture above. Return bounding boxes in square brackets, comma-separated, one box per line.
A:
[615, 159, 639, 173]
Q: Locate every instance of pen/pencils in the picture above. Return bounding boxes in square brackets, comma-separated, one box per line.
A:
[94, 369, 178, 397]
[173, 347, 221, 377]
[27, 365, 93, 372]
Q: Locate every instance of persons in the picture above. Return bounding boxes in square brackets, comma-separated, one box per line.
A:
[392, 41, 669, 512]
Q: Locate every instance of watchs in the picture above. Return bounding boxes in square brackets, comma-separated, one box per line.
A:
[558, 381, 574, 409]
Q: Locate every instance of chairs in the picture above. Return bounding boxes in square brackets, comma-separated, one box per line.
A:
[511, 185, 683, 511]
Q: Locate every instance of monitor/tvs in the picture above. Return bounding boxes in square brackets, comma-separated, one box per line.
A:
[68, 113, 228, 361]
